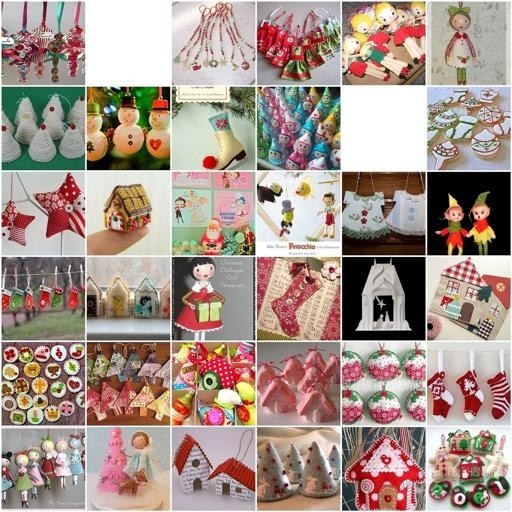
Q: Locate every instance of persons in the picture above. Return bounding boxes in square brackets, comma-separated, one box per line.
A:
[4, 172, 510, 510]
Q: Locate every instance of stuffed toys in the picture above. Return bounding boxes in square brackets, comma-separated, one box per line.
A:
[445, 2, 478, 85]
[3, 173, 511, 510]
[254, 2, 428, 168]
[86, 88, 170, 162]
